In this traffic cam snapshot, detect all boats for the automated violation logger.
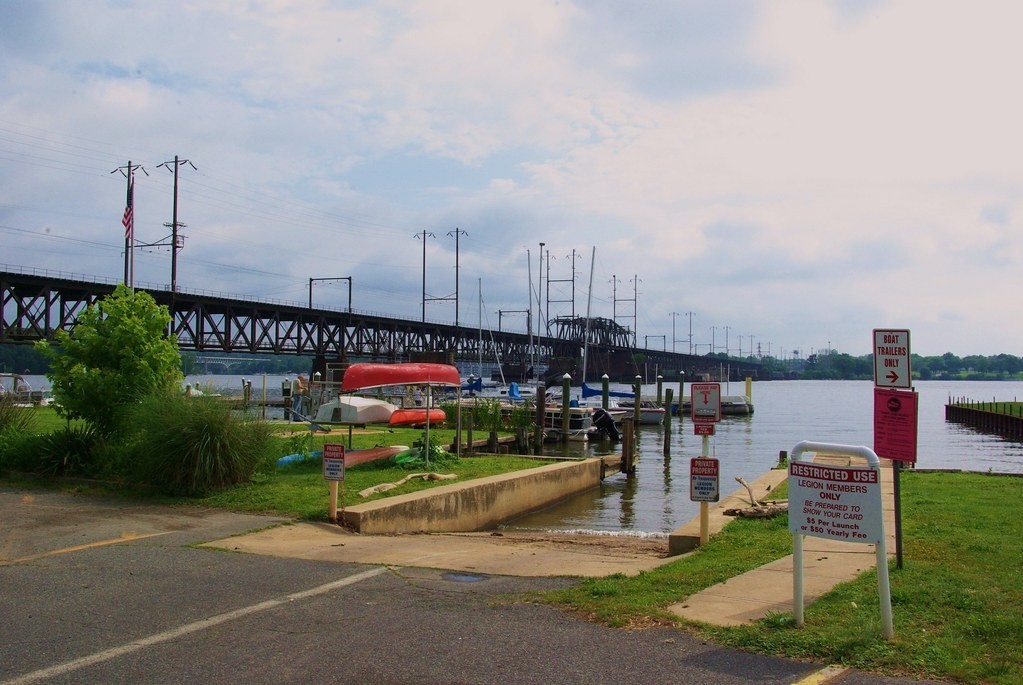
[389,408,447,427]
[337,362,461,395]
[430,246,755,442]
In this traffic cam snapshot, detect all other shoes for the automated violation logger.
[293,419,303,422]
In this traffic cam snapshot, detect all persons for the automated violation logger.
[414,386,425,406]
[293,375,308,421]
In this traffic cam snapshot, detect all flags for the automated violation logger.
[122,176,135,239]
